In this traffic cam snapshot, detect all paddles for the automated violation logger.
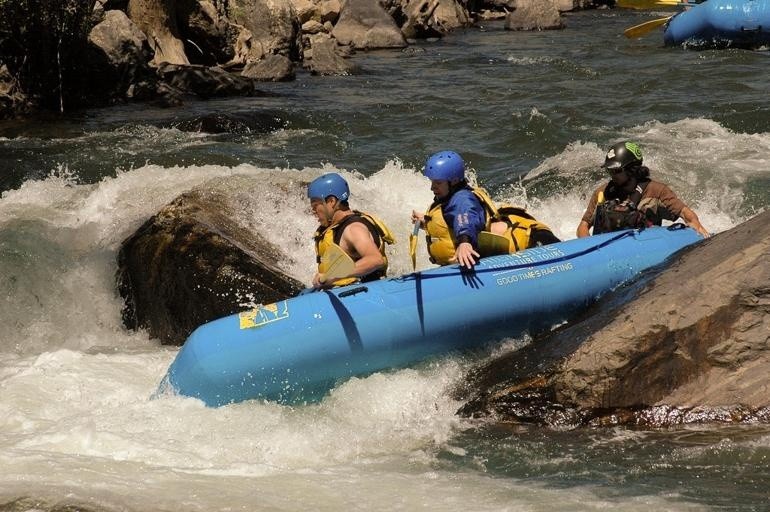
[309,244,355,289]
[408,219,421,269]
[625,17,670,40]
[617,0,697,10]
[478,231,509,256]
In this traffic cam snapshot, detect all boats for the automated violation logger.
[663,1,770,47]
[147,223,704,408]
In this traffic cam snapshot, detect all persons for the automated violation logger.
[305,171,390,292]
[489,201,562,257]
[574,139,712,244]
[411,149,491,269]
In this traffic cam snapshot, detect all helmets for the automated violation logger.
[306,173,352,204]
[424,150,466,183]
[599,139,643,168]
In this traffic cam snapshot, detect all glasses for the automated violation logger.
[607,169,623,177]
